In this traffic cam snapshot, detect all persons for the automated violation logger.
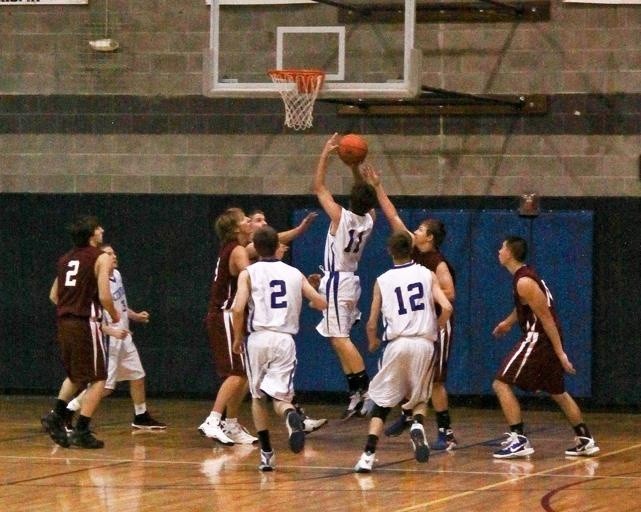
[197,206,290,446]
[354,229,454,473]
[244,210,327,435]
[62,244,168,436]
[490,234,602,459]
[40,212,121,449]
[359,162,459,452]
[231,225,328,471]
[314,130,375,424]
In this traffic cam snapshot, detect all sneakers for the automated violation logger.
[492,433,535,459]
[197,390,457,473]
[42,410,103,450]
[131,412,166,429]
[564,436,601,456]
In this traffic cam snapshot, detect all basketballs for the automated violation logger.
[338,135,367,163]
